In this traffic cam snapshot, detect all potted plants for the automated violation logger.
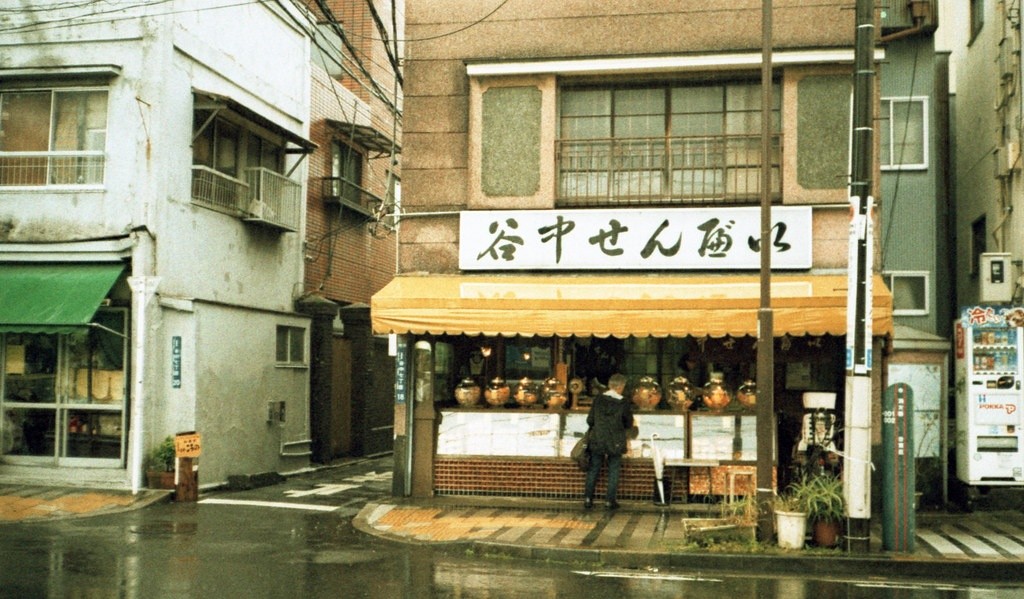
[143,443,160,489]
[773,494,807,550]
[153,435,175,490]
[785,471,844,547]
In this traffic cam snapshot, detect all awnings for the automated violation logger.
[0,262,133,345]
[369,273,897,340]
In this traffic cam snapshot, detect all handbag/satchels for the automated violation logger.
[571,427,591,472]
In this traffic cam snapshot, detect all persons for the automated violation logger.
[582,373,640,511]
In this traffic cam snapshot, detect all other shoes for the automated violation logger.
[605,499,620,510]
[584,497,593,508]
